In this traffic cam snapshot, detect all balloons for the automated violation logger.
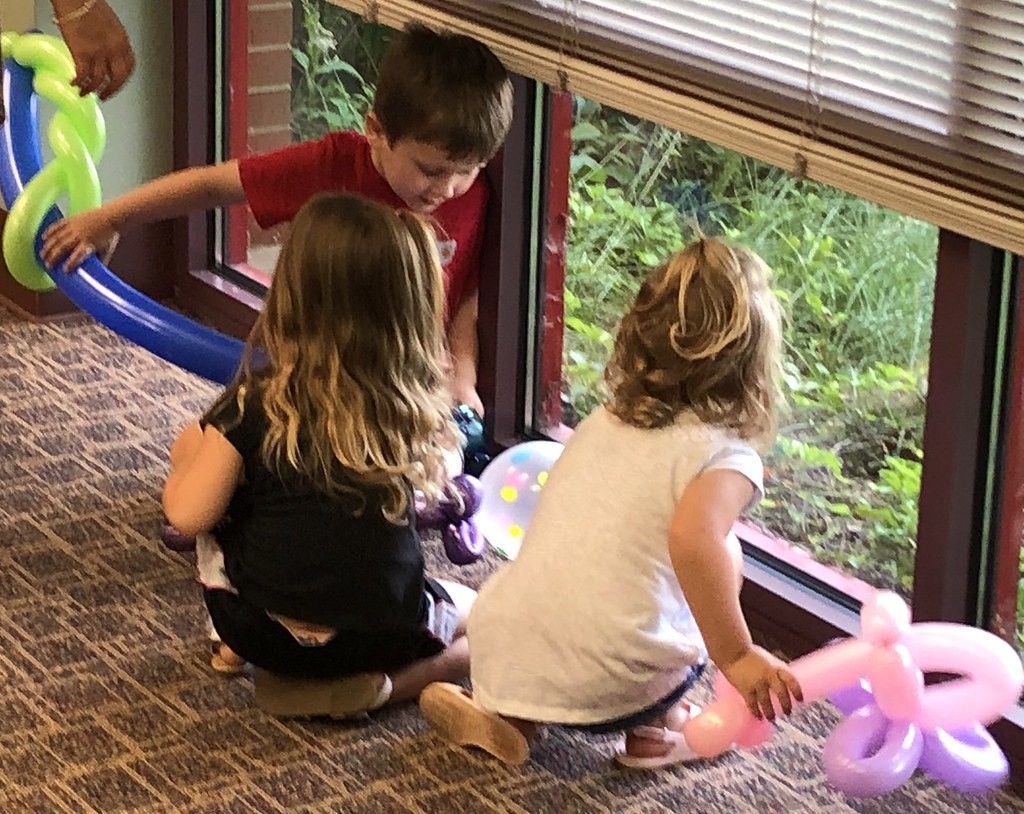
[411,475,484,566]
[473,441,565,562]
[0,29,267,388]
[685,588,1024,799]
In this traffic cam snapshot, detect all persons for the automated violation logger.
[162,192,478,717]
[48,0,136,101]
[418,237,804,769]
[40,26,513,423]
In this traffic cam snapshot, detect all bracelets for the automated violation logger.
[52,0,97,25]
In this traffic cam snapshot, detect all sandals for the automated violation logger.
[210,629,254,675]
[618,700,734,769]
[253,672,393,718]
[420,681,531,766]
